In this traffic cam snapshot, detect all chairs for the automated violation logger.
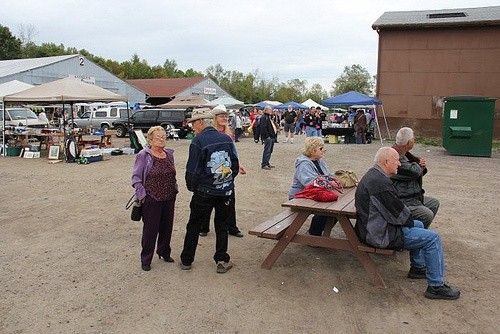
[99,135,111,148]
[74,135,84,150]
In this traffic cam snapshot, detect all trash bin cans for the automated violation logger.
[443,96,496,158]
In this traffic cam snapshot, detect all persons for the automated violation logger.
[231,110,243,143]
[131,126,178,272]
[289,136,341,247]
[31,108,82,124]
[387,127,440,229]
[354,147,460,296]
[180,107,240,273]
[250,107,264,143]
[200,104,246,238]
[259,105,276,170]
[294,106,375,144]
[283,105,297,144]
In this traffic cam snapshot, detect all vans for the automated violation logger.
[94,107,133,128]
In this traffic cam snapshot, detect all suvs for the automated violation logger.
[112,109,188,138]
[0,107,48,135]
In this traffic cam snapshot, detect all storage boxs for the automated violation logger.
[81,149,102,162]
[6,147,21,156]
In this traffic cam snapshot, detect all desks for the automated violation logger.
[42,129,59,136]
[323,128,355,143]
[35,134,65,152]
[260,176,387,290]
[13,133,34,149]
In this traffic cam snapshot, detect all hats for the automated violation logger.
[187,108,214,123]
[210,104,228,115]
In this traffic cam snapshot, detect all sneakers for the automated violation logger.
[425,282,460,299]
[216,260,233,273]
[407,266,427,279]
[180,256,192,269]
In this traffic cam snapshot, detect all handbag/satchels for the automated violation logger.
[335,169,360,188]
[312,173,345,194]
[125,194,142,221]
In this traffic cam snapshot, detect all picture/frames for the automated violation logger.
[48,145,61,160]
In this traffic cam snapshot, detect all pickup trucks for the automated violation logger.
[68,110,118,130]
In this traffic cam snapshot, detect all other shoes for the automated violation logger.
[200,232,207,236]
[262,165,271,170]
[268,164,275,168]
[229,229,243,237]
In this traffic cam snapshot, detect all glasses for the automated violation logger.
[317,147,324,151]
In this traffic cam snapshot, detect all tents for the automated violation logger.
[0,77,131,162]
[157,96,260,111]
[256,91,391,146]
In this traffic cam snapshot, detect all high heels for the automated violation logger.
[158,252,174,262]
[141,264,150,270]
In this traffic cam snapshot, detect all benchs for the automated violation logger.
[248,203,300,238]
